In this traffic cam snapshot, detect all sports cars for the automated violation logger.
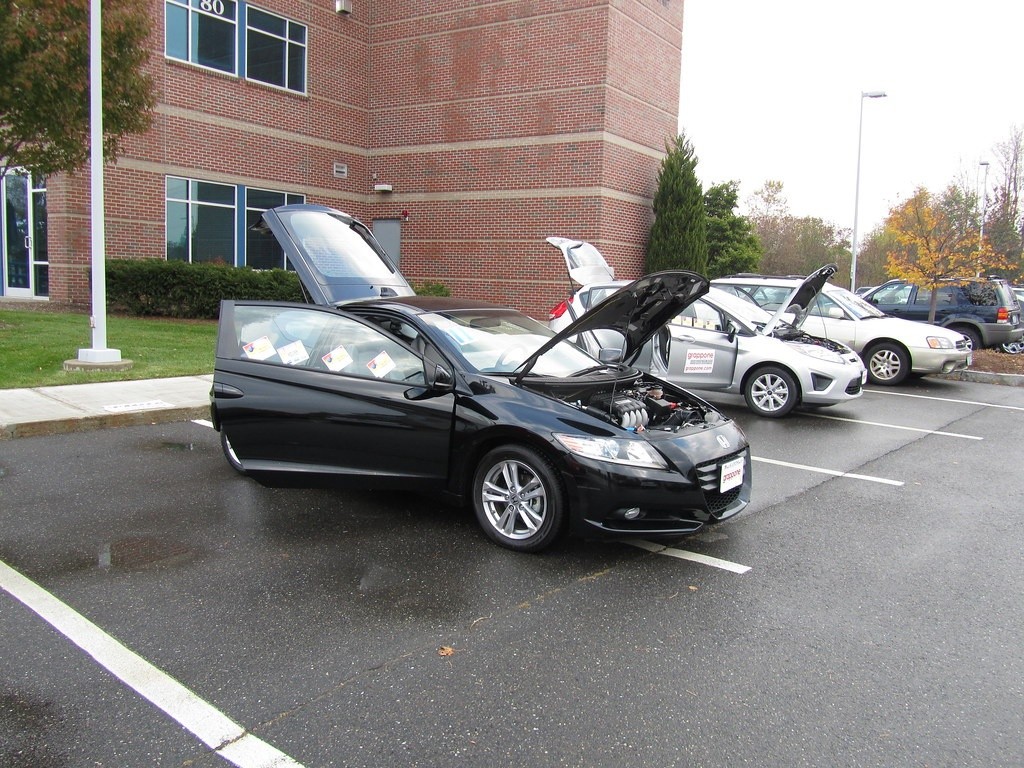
[207,203,754,552]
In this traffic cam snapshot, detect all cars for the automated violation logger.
[542,230,870,418]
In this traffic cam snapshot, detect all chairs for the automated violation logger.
[347,342,399,376]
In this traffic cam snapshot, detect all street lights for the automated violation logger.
[850,90,886,291]
[975,160,990,277]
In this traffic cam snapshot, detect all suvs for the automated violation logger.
[824,276,1024,349]
[704,271,975,384]
